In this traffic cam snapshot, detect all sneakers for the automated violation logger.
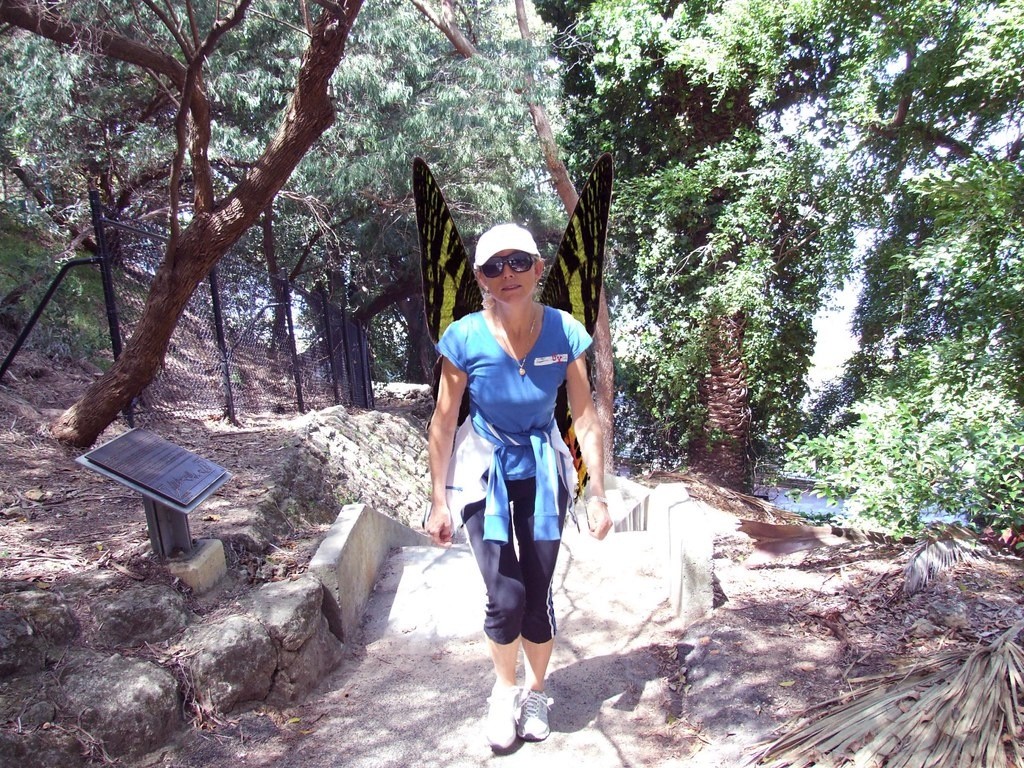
[486,682,520,752]
[517,688,551,741]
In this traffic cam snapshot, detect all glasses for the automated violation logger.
[482,252,533,278]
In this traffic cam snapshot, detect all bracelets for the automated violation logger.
[586,496,609,506]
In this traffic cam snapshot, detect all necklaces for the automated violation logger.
[490,303,538,375]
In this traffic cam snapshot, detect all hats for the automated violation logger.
[475,224,540,267]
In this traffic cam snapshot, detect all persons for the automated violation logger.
[425,223,614,750]
